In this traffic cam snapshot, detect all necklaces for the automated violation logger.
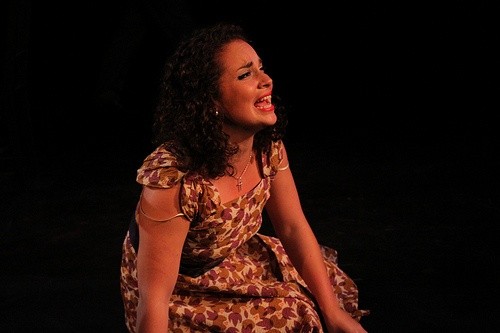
[226,150,254,192]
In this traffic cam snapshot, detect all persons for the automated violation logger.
[119,23,372,333]
